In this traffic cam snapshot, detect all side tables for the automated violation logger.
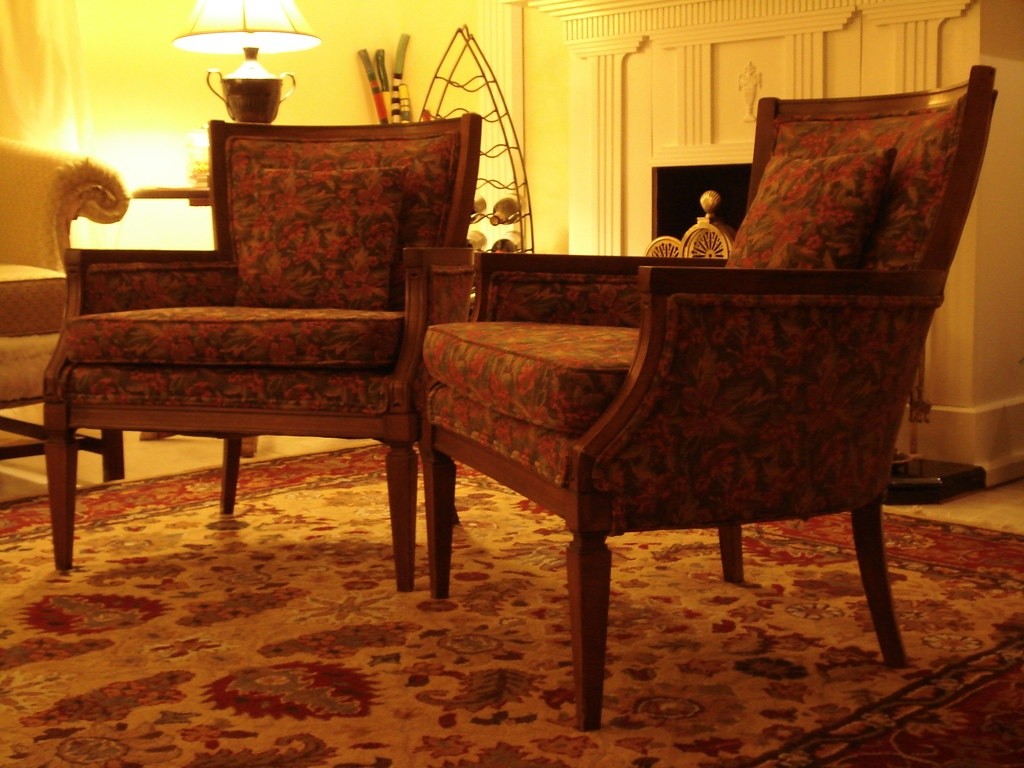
[128,185,258,459]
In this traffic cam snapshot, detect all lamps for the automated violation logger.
[169,0,322,124]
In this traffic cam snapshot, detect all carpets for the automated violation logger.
[0,444,1024,768]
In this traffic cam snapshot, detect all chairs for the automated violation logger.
[43,112,483,593]
[419,63,998,732]
[0,134,126,483]
